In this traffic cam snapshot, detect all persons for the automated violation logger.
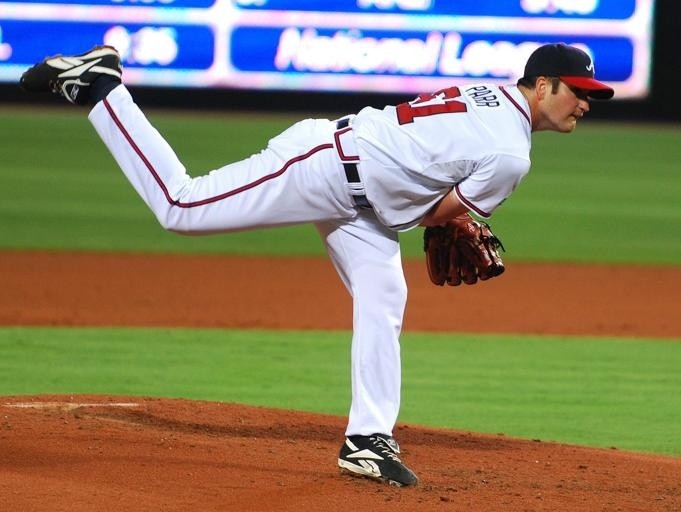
[20,45,614,488]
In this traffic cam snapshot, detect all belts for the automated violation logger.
[335,116,372,212]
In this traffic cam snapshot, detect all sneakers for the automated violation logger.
[18,44,124,107]
[337,433,420,488]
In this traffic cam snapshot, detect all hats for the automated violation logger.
[523,42,615,99]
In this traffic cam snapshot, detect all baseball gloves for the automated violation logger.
[424,212,505,286]
